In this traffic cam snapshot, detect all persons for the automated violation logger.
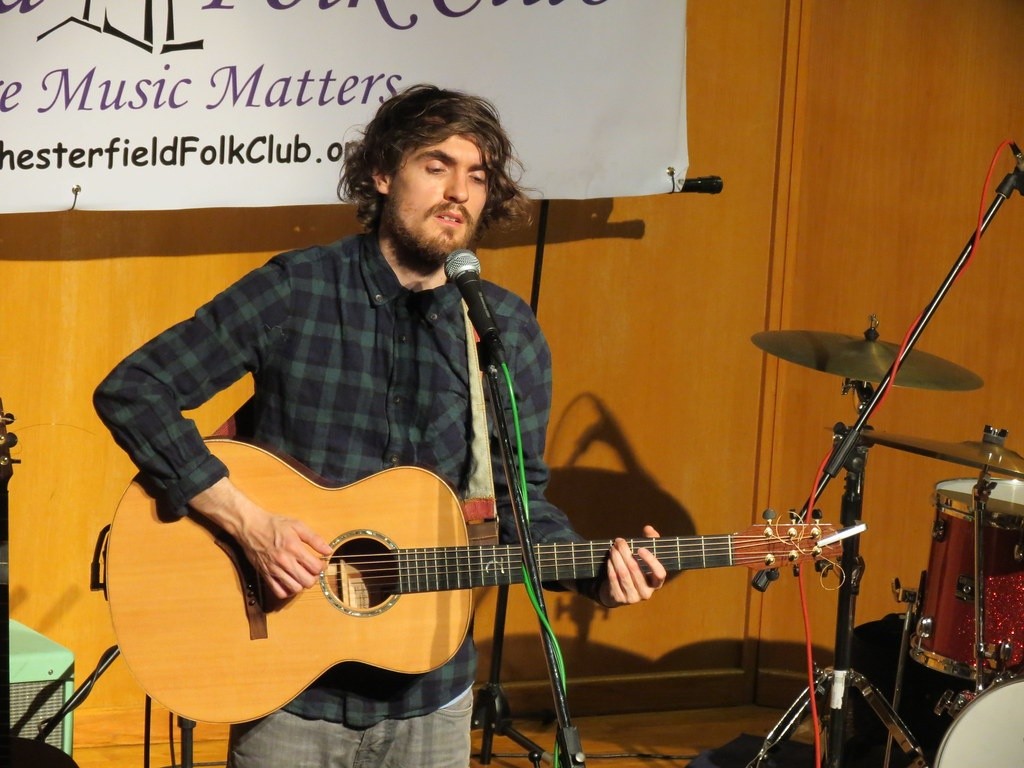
[91,84,665,768]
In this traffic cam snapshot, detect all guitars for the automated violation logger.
[89,435,867,724]
[1,399,82,768]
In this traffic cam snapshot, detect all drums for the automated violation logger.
[931,679,1023,768]
[909,478,1024,683]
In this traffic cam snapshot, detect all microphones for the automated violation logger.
[444,247,508,365]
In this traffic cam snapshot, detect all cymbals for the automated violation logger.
[749,329,984,393]
[824,424,1023,480]
